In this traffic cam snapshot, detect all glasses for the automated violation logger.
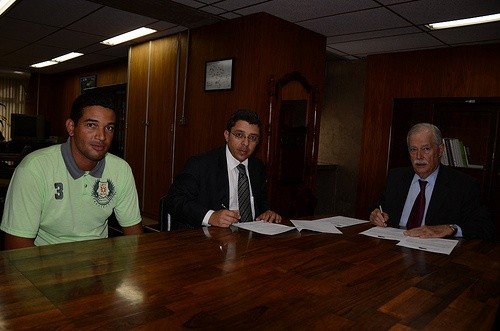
[228,130,260,143]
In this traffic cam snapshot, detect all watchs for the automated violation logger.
[449,225,457,236]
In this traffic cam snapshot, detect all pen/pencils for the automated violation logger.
[222,204,243,223]
[379,205,387,228]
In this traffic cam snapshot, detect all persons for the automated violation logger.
[0,91,142,250]
[368,123,496,241]
[164,108,282,228]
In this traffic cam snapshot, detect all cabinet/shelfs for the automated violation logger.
[77,82,126,157]
[388,96,500,204]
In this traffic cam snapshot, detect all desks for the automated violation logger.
[0,229,500,331]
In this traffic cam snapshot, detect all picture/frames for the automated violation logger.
[203,57,236,91]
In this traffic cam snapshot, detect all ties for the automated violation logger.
[406,179,428,232]
[237,164,253,222]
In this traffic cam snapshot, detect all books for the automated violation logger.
[441,138,469,168]
